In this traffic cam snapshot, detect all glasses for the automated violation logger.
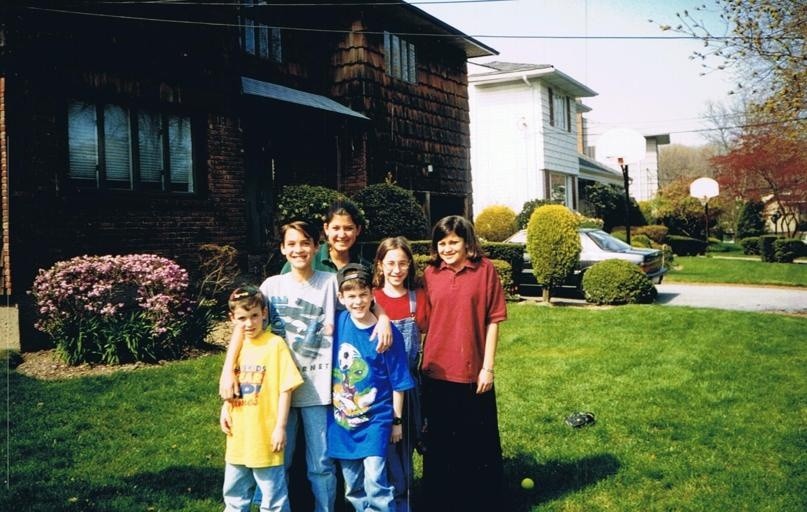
[381,260,412,268]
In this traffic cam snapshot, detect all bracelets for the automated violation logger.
[482,368,495,373]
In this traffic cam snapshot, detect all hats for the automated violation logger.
[336,263,375,308]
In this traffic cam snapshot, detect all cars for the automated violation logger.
[499,224,668,297]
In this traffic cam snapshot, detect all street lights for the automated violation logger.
[689,175,721,236]
[594,128,651,244]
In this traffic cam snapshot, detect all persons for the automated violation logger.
[219,284,304,512]
[327,263,414,511]
[369,237,427,493]
[218,221,393,512]
[418,216,507,507]
[280,202,374,285]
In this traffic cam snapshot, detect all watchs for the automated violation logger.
[393,418,403,425]
